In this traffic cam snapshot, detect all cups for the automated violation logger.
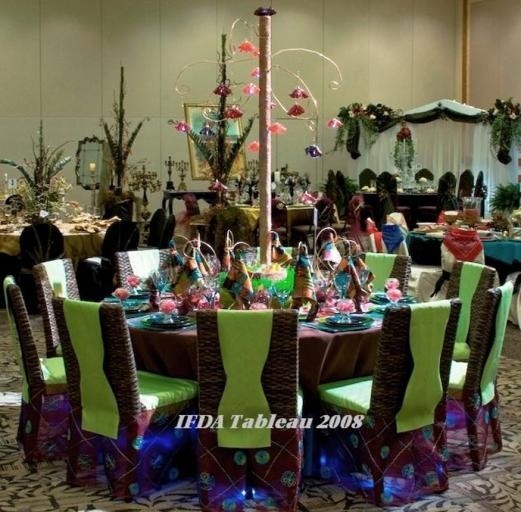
[358,270,369,287]
[334,273,352,298]
[199,278,218,309]
[150,269,170,302]
[274,280,293,309]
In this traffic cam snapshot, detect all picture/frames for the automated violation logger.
[184,103,248,180]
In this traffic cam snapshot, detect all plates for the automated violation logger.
[324,315,374,328]
[128,291,150,299]
[371,293,416,304]
[376,305,409,315]
[112,302,151,315]
[140,314,196,329]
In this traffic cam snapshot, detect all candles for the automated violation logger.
[90,163,96,171]
